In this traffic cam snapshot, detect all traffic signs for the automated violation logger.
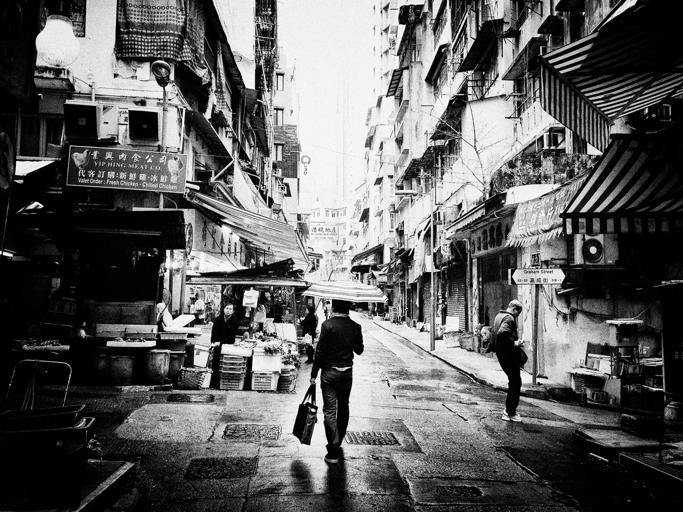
[507,268,566,286]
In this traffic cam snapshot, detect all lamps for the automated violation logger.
[148,56,174,212]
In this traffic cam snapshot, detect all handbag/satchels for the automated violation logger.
[486,333,498,354]
[292,384,318,445]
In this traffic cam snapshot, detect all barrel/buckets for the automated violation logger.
[193,345,209,368]
[170,349,187,379]
[146,348,170,378]
[665,403,682,439]
[570,369,585,393]
[110,353,132,380]
[584,373,604,400]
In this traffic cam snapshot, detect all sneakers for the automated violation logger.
[324,451,339,463]
[502,407,523,422]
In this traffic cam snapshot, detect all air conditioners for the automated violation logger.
[565,230,620,268]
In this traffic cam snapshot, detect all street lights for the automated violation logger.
[148,59,170,208]
[395,188,437,351]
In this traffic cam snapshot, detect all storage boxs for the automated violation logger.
[181,334,297,394]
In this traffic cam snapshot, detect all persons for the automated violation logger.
[299,305,316,364]
[321,300,332,322]
[492,299,526,422]
[308,300,365,466]
[265,297,283,324]
[210,301,237,389]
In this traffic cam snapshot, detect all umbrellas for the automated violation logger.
[298,276,389,306]
